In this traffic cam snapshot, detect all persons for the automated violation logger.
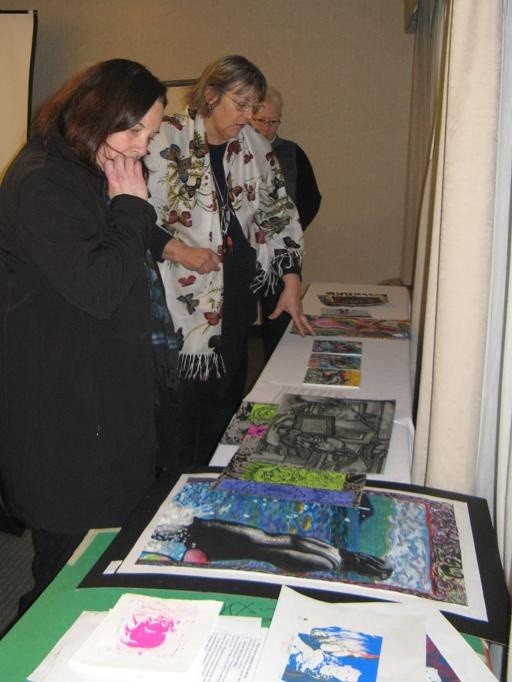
[222,83,327,410]
[1,57,180,606]
[143,51,318,463]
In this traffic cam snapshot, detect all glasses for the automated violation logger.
[209,83,265,114]
[252,117,282,127]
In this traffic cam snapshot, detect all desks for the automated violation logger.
[150,279,417,682]
